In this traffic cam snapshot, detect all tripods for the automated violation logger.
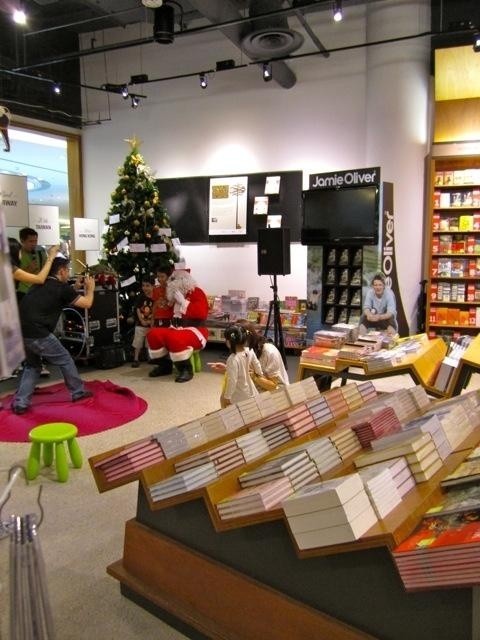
[263,275,288,371]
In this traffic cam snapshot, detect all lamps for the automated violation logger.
[332,0,344,26]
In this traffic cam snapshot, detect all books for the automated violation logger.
[435,168,480,184]
[359,332,428,374]
[299,323,359,370]
[431,282,480,301]
[433,214,480,230]
[433,190,480,206]
[432,257,480,276]
[93,396,262,550]
[263,396,390,550]
[391,392,480,595]
[210,295,306,324]
[432,235,480,253]
[428,331,478,347]
[430,307,480,325]
[428,347,469,392]
[289,372,429,395]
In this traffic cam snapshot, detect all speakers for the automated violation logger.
[257,227,291,276]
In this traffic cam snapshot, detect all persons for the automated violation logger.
[145,260,210,383]
[7,238,60,294]
[358,276,399,332]
[18,227,47,294]
[130,275,156,368]
[207,323,289,395]
[221,325,263,410]
[10,257,95,416]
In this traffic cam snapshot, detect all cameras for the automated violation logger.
[80,269,95,283]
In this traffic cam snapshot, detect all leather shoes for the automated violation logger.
[149,366,172,377]
[14,405,26,414]
[175,369,193,382]
[72,391,92,401]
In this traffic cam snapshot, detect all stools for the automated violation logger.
[22,422,83,484]
[180,347,205,376]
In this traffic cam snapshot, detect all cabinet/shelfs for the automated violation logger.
[87,376,480,639]
[296,325,479,396]
[321,241,365,332]
[427,152,480,338]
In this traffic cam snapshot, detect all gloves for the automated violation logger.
[166,289,174,305]
[174,290,185,304]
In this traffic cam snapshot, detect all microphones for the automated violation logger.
[31,248,36,261]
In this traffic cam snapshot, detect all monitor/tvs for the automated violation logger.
[301,181,379,246]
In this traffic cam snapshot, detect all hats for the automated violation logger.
[172,262,191,273]
